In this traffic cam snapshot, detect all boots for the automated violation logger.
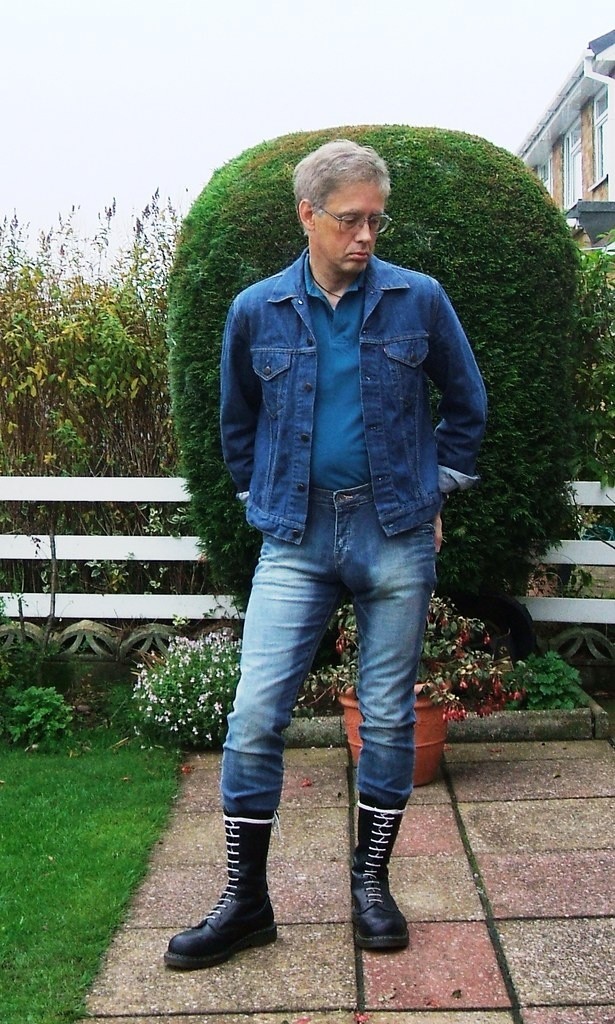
[163,810,281,968]
[351,792,410,948]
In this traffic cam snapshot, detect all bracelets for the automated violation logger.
[442,494,450,503]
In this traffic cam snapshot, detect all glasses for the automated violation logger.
[314,203,393,235]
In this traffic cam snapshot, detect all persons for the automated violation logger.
[163,138,487,967]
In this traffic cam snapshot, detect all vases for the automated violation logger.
[338,694,447,787]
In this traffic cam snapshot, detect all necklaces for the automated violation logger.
[308,260,341,298]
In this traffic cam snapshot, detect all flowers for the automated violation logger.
[127,630,240,744]
[302,594,524,721]
